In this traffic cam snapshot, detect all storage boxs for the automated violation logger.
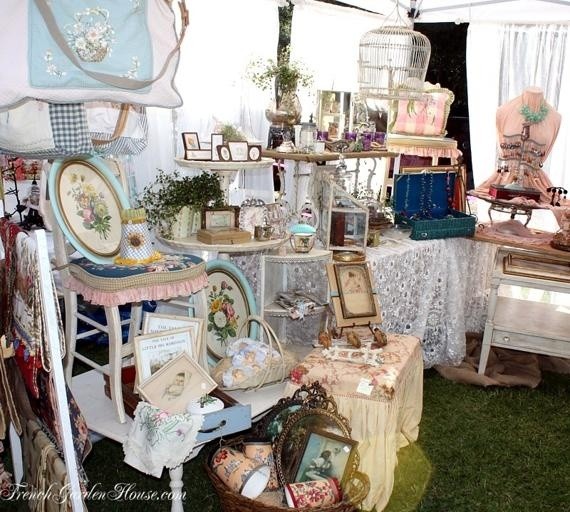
[393,173,476,241]
[1,2,190,159]
[185,149,211,161]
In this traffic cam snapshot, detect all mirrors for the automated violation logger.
[281,414,344,481]
[267,405,301,442]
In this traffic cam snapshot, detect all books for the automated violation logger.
[132,311,218,413]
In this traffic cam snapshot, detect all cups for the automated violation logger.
[289,232,316,254]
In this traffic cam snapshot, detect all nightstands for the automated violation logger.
[477,246,569,376]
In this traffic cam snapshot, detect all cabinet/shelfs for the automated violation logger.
[261,249,333,361]
[231,220,570,370]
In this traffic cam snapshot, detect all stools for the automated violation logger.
[291,330,423,512]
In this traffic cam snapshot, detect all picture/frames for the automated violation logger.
[49,153,130,265]
[133,312,204,394]
[136,351,218,415]
[276,391,360,486]
[189,260,258,367]
[211,133,223,161]
[182,132,201,149]
[134,326,198,403]
[217,145,230,162]
[248,145,261,161]
[202,206,240,229]
[228,140,248,162]
[256,380,328,442]
[289,426,358,502]
[326,261,382,328]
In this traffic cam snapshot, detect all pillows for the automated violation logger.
[387,85,455,137]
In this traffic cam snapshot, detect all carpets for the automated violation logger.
[0,368,570,512]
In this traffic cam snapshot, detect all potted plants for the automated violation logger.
[134,168,227,239]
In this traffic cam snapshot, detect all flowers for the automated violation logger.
[248,45,316,91]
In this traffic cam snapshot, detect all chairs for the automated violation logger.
[71,360,299,511]
[51,154,209,426]
[382,79,463,204]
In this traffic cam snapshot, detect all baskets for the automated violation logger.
[206,444,370,512]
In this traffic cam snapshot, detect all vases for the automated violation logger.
[266,92,303,151]
